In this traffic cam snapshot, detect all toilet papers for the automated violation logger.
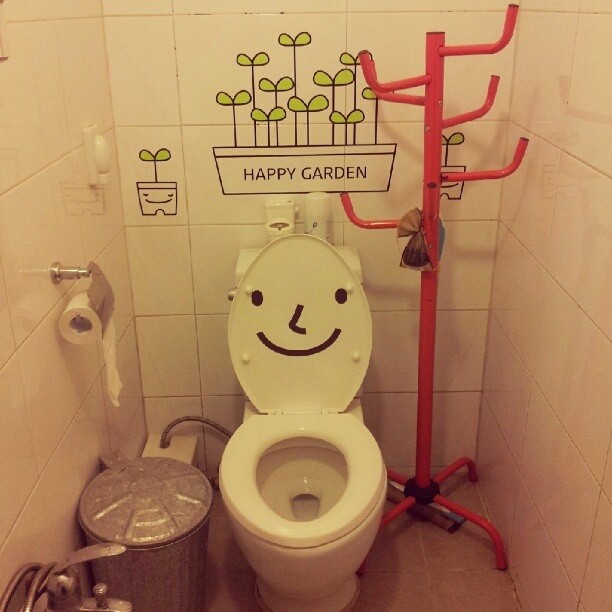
[58,292,123,411]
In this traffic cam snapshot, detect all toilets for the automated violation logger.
[218,233,388,612]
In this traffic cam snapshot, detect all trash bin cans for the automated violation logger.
[79,448,212,612]
